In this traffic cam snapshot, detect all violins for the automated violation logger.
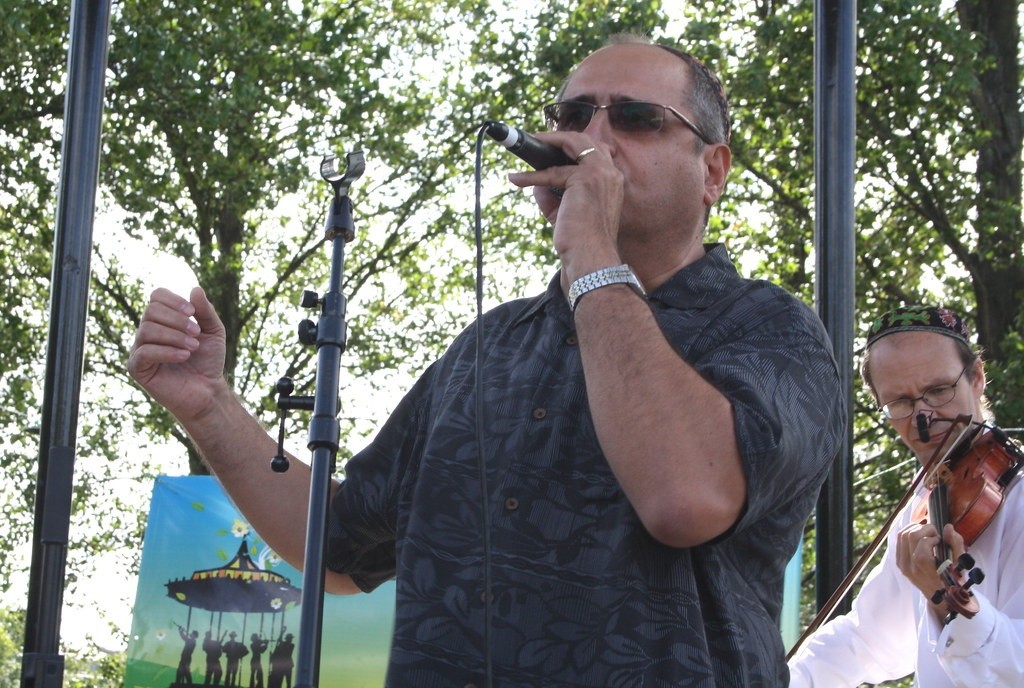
[910,426,1020,625]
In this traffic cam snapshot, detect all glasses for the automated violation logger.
[879,359,973,420]
[544,101,712,146]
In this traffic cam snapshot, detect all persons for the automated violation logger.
[127,36,847,688]
[786,306,1023,688]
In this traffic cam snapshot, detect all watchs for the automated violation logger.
[567,264,646,311]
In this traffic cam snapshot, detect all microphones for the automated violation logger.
[483,121,580,198]
[917,413,931,443]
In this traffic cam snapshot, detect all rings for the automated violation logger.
[575,148,598,164]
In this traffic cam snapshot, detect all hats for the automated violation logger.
[866,305,971,346]
